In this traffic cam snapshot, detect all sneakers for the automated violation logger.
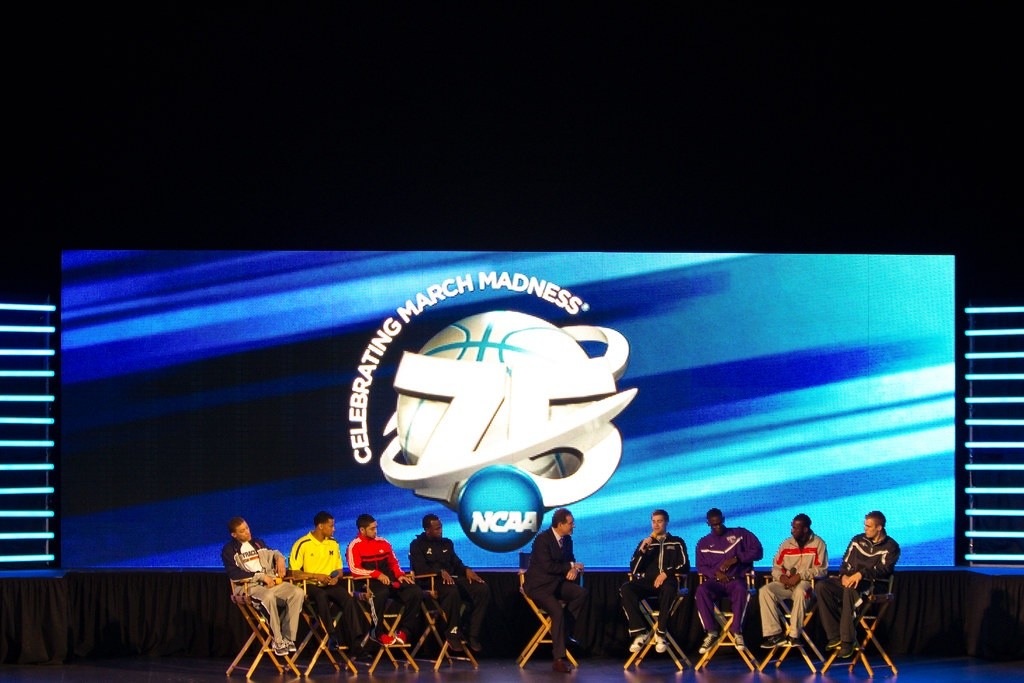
[446,632,462,652]
[655,633,666,652]
[327,638,340,651]
[378,634,396,645]
[272,642,289,656]
[760,634,786,648]
[396,631,407,644]
[283,638,296,651]
[826,637,841,650]
[350,648,372,660]
[629,631,648,653]
[469,636,481,651]
[841,638,857,658]
[698,635,717,654]
[787,637,802,646]
[734,633,744,650]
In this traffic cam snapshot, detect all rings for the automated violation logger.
[787,586,788,587]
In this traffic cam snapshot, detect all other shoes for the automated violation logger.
[552,659,571,672]
[567,629,580,642]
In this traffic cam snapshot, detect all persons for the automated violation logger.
[694,509,763,654]
[815,511,900,657]
[623,510,690,652]
[288,512,372,659]
[524,509,588,672]
[410,513,489,651]
[758,514,830,648]
[223,517,304,656]
[346,514,422,645]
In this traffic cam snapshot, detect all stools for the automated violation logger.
[516,552,583,668]
[226,552,478,679]
[623,569,898,678]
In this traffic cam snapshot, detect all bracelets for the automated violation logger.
[734,556,737,558]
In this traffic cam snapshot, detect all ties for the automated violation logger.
[560,539,563,548]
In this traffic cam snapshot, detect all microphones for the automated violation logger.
[717,577,736,581]
[388,581,401,589]
[643,531,657,549]
[259,579,283,586]
[316,570,338,586]
[785,567,797,589]
